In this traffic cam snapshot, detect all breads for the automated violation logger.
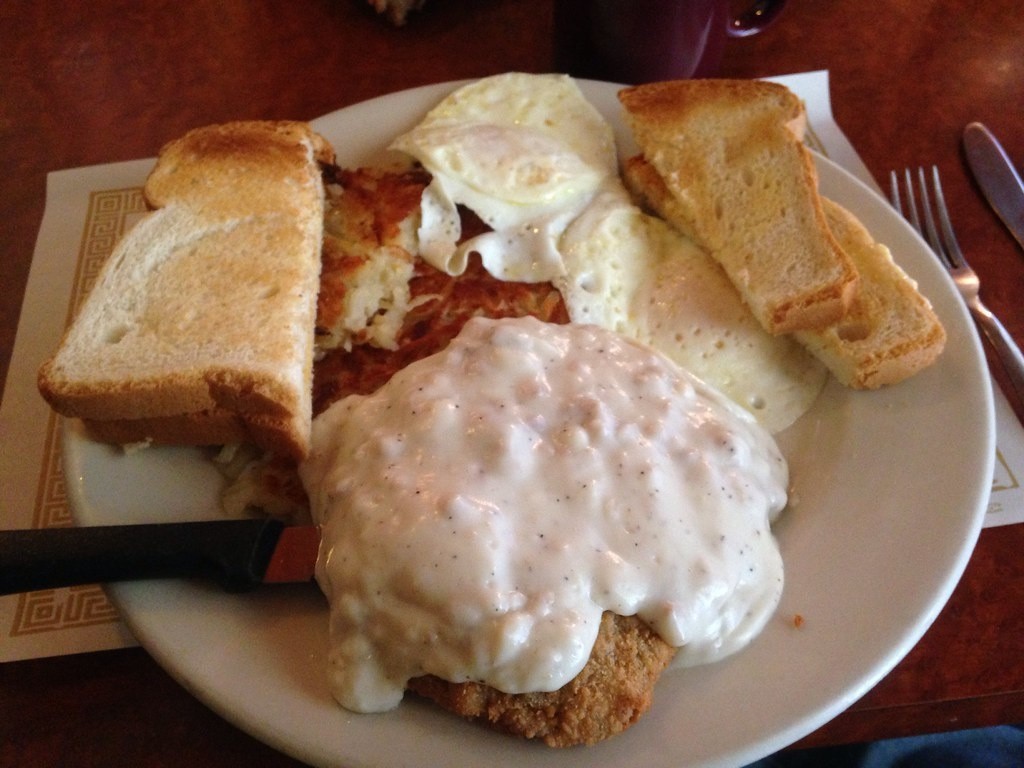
[35,79,945,458]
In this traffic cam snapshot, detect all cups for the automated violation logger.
[552,0,713,88]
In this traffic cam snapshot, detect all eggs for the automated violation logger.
[301,73,827,713]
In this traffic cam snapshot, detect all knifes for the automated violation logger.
[1,520,322,595]
[963,122,1024,250]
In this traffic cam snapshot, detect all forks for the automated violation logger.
[888,167,1024,404]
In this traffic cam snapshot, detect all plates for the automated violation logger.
[63,73,995,768]
